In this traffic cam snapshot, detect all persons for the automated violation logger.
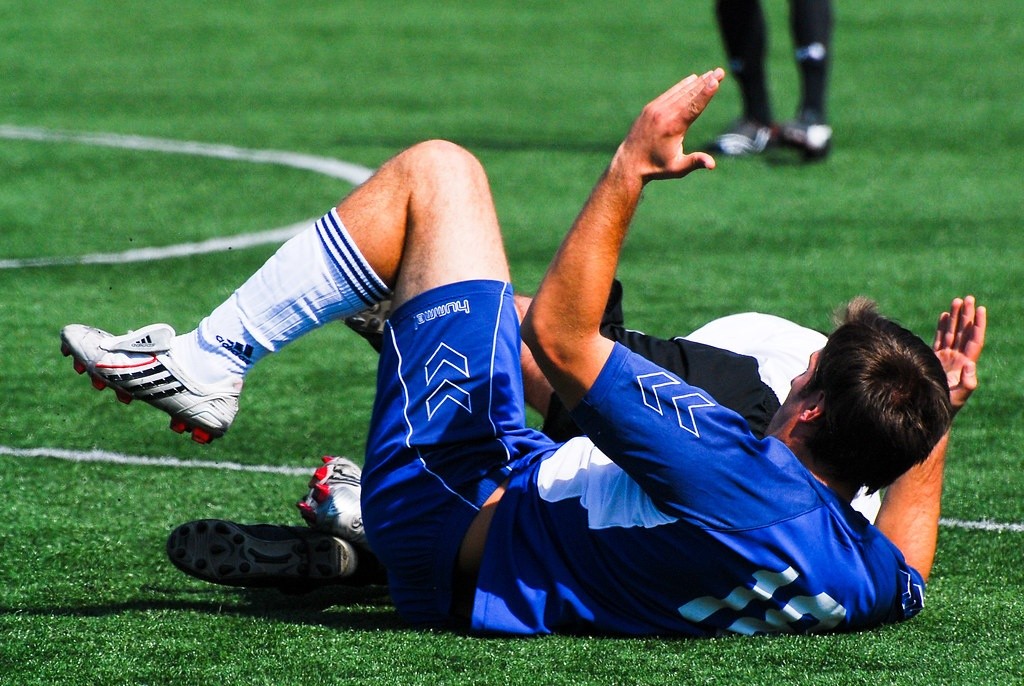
[299,280,882,588]
[714,0,834,162]
[59,67,987,640]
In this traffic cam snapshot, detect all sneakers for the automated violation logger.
[166,517,362,584]
[341,287,396,354]
[295,454,365,544]
[59,322,243,445]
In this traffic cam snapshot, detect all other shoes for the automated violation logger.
[781,119,833,155]
[707,121,780,156]
